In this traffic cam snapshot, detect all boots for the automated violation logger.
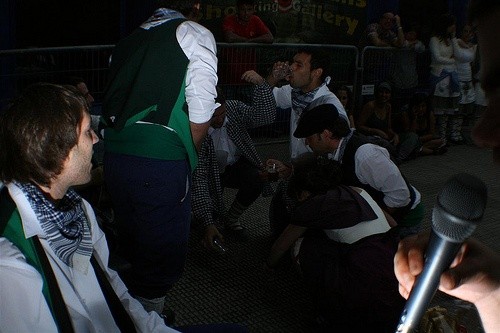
[223,196,249,240]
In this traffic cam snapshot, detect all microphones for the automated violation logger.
[394,173,486,333]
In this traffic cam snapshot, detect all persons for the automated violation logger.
[222,0,273,106]
[267,104,425,244]
[0,81,247,333]
[264,156,416,322]
[392,0,500,333]
[266,0,491,160]
[265,44,355,161]
[55,78,112,228]
[189,68,277,250]
[97,1,218,328]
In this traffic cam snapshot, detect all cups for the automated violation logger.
[214,238,230,258]
[273,64,292,80]
[266,163,278,181]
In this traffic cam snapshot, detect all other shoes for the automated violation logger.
[451,138,464,146]
[160,309,175,326]
[439,144,448,154]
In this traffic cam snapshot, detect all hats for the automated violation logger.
[293,104,340,138]
[377,82,392,90]
